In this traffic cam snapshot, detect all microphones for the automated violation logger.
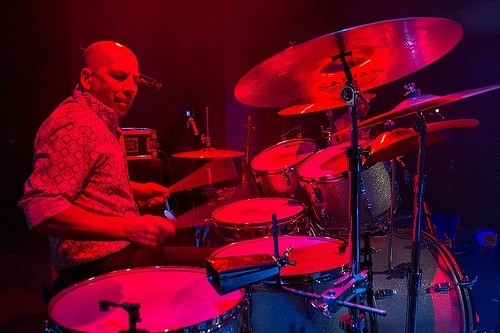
[187,113,198,136]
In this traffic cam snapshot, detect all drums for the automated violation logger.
[359,226,477,333]
[212,198,309,243]
[251,138,318,197]
[45,266,250,333]
[298,139,400,234]
[207,235,350,333]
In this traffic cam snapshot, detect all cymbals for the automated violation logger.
[234,17,463,107]
[325,82,497,132]
[362,118,478,165]
[172,147,245,159]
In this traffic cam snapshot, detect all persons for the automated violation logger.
[18,40,217,286]
[331,94,397,236]
[159,105,211,180]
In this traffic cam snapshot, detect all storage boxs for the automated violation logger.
[121,128,157,160]
